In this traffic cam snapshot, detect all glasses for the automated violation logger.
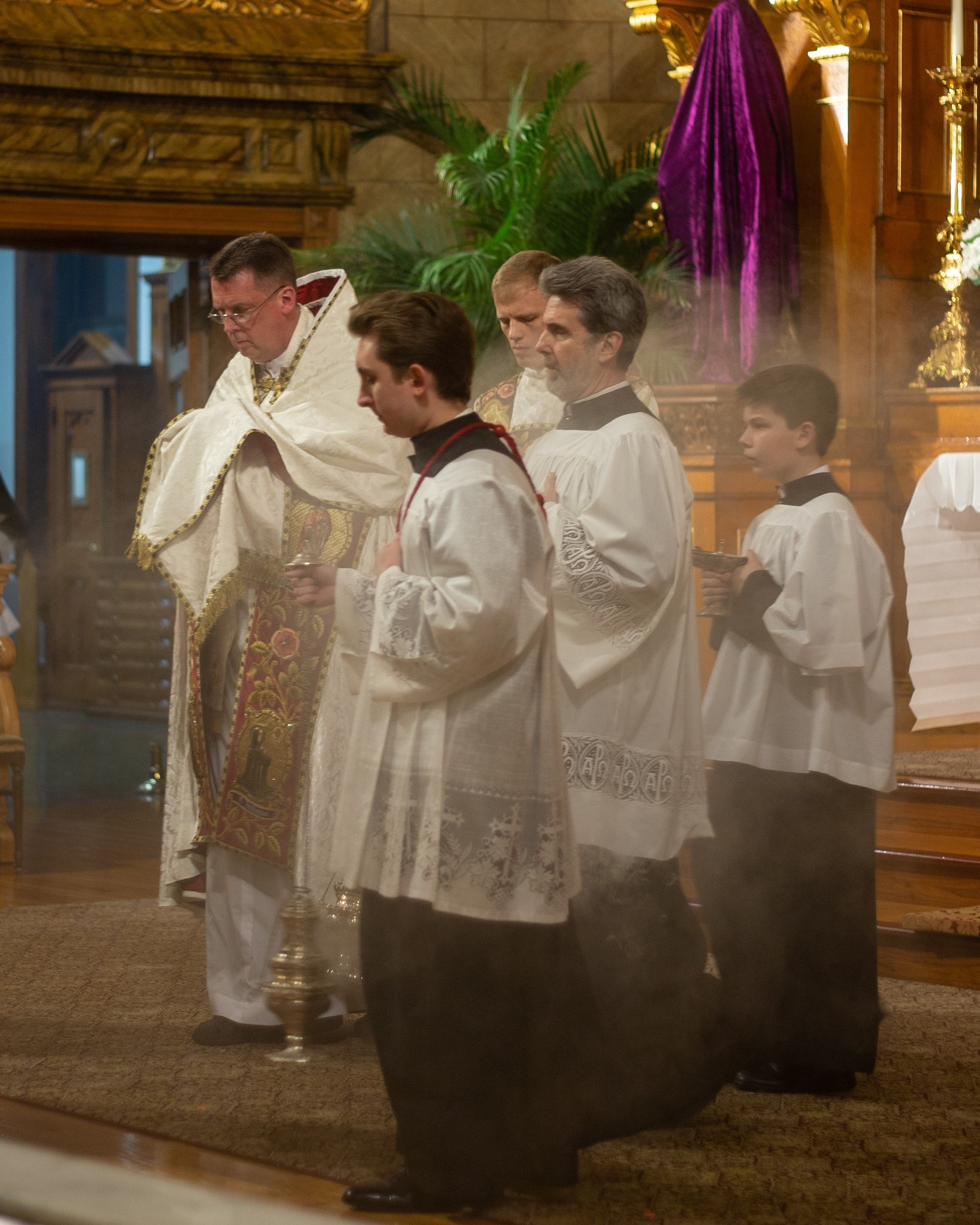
[207,285,286,324]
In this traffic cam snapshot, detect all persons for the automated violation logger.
[189,224,412,1051]
[283,287,599,1211]
[695,361,893,1097]
[463,247,662,480]
[521,254,720,1147]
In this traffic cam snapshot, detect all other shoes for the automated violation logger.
[737,1071,857,1093]
[191,1014,344,1045]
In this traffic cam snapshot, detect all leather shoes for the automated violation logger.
[343,1165,463,1215]
